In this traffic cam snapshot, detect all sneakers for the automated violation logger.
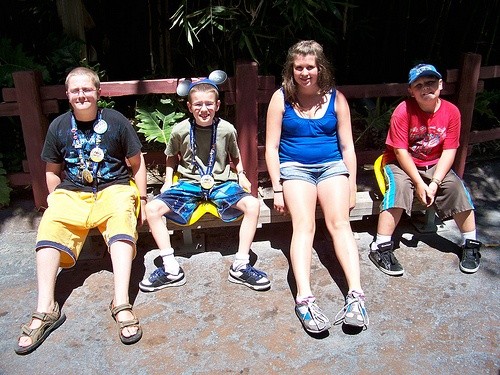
[138,266,187,292]
[295,296,332,333]
[341,291,366,328]
[458,240,482,272]
[368,241,404,276]
[228,263,270,290]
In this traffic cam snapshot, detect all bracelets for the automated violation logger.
[274,190,283,193]
[430,177,441,187]
[236,169,247,177]
[139,194,148,202]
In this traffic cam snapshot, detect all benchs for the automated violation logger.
[76,171,450,261]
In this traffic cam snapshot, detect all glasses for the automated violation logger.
[69,88,97,95]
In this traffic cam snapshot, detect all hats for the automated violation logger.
[408,64,442,85]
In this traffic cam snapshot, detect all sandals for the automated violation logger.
[14,303,67,353]
[109,300,142,344]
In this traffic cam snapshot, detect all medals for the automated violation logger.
[81,168,93,184]
[200,174,214,190]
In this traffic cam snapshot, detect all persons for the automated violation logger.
[10,66,150,356]
[264,39,371,333]
[135,80,273,292]
[368,64,484,277]
